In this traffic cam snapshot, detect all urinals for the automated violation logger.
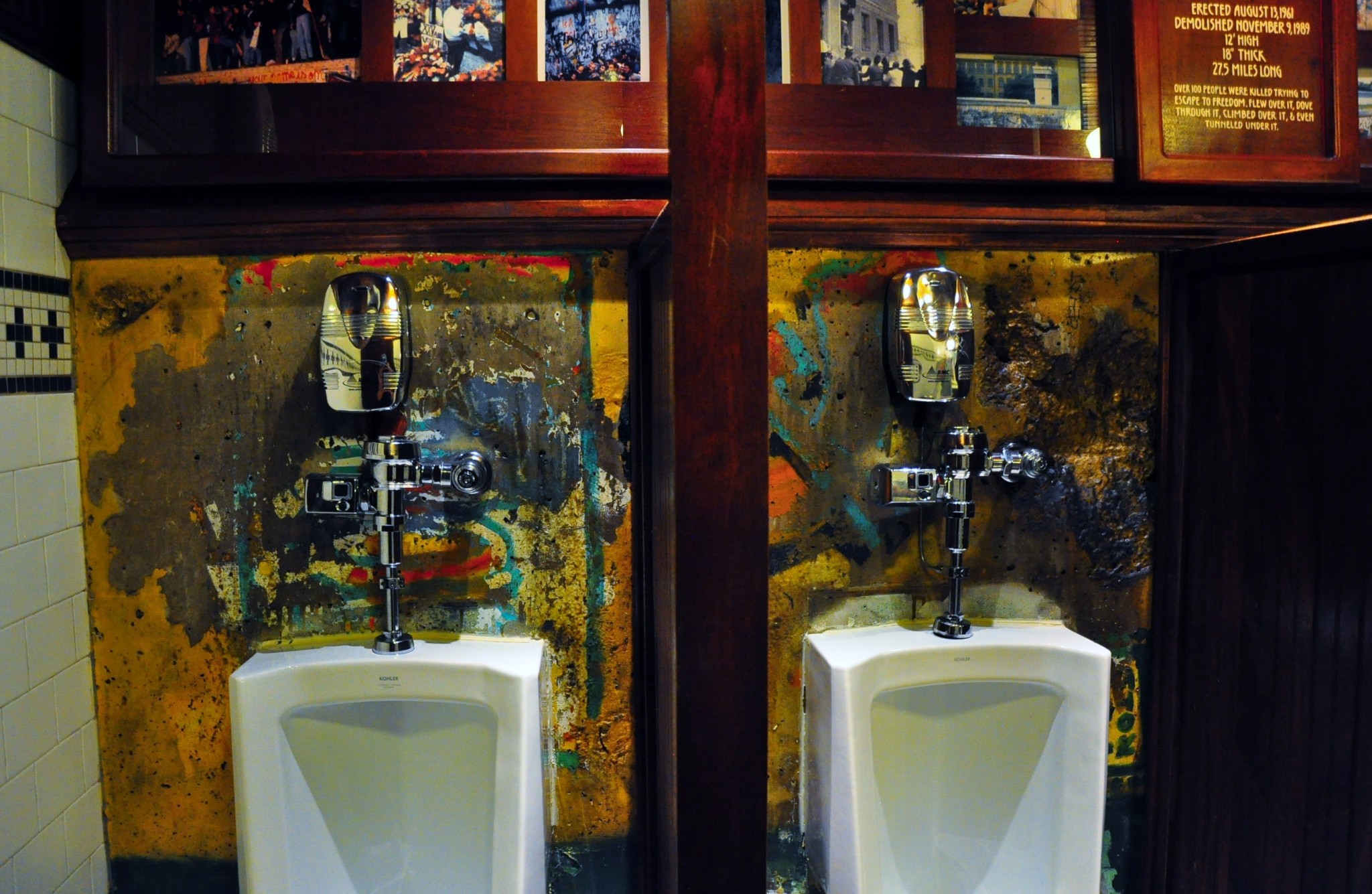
[223,631,550,894]
[803,618,1110,893]
